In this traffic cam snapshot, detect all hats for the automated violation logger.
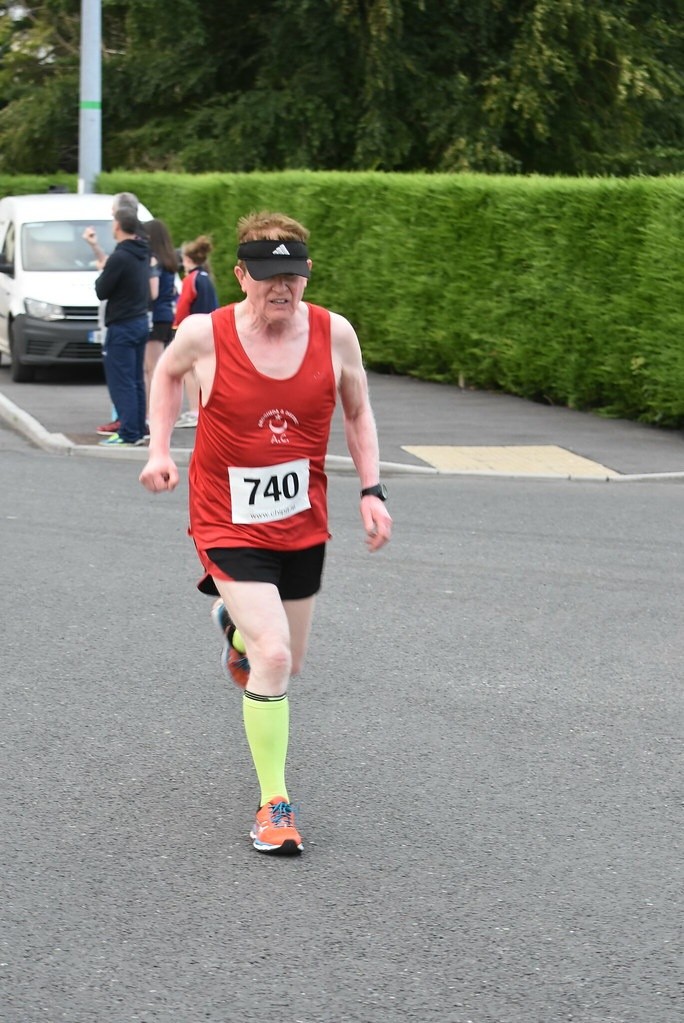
[237,241,310,280]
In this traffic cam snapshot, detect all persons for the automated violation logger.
[82,192,180,437]
[173,236,217,428]
[139,210,393,852]
[94,207,150,447]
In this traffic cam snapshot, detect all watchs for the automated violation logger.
[360,483,389,502]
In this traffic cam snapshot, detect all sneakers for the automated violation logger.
[99,433,145,447]
[96,419,150,439]
[251,796,304,854]
[211,598,250,691]
[174,412,198,428]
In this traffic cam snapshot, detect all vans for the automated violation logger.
[0,195,184,383]
